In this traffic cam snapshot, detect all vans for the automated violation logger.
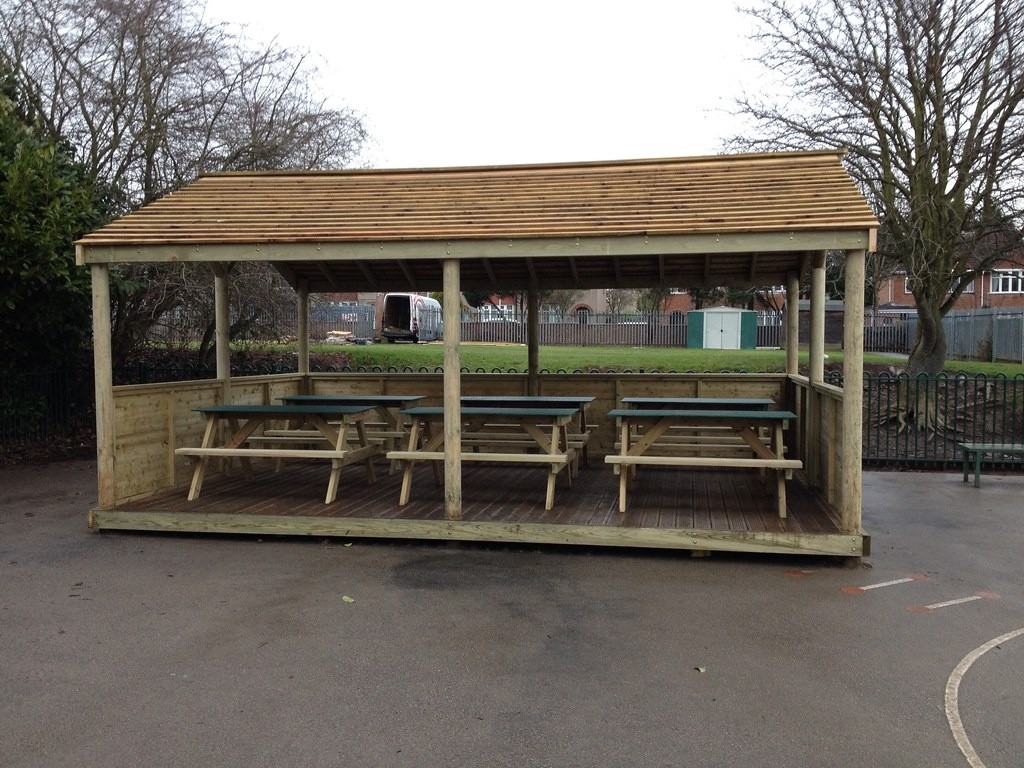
[379,291,445,343]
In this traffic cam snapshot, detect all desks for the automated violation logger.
[397,406,580,510]
[275,395,428,474]
[186,405,387,505]
[621,396,777,480]
[605,409,803,518]
[462,395,597,477]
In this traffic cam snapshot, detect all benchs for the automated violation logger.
[265,430,407,438]
[304,422,427,428]
[244,436,388,445]
[462,432,589,440]
[464,423,599,430]
[618,435,772,444]
[386,450,569,463]
[958,442,1024,488]
[629,426,769,432]
[174,447,349,458]
[424,439,585,449]
[605,455,802,468]
[614,443,787,453]
